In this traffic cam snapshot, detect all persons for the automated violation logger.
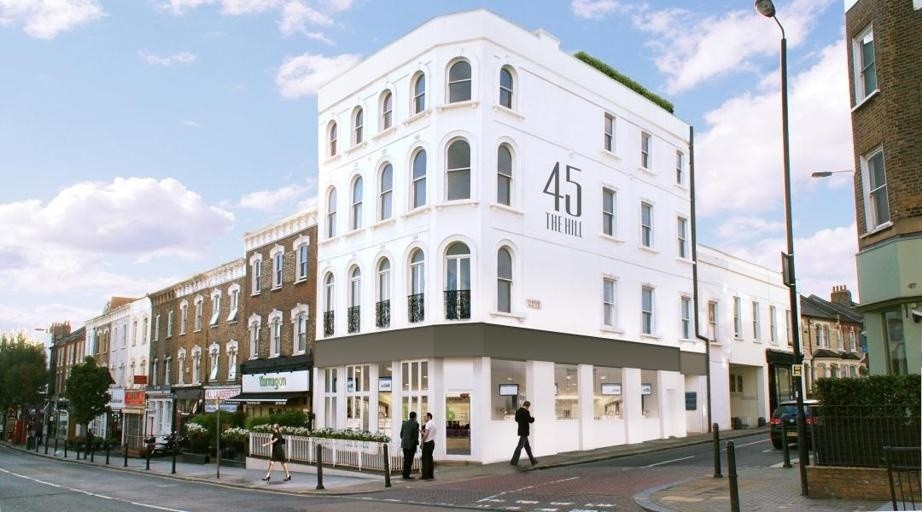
[510,400,539,467]
[400,411,419,479]
[418,413,436,480]
[262,424,293,483]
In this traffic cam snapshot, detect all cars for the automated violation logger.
[769,399,821,450]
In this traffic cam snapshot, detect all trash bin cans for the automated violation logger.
[733,417,741,429]
[759,417,765,426]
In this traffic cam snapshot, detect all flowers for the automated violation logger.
[221,426,249,449]
[185,423,209,441]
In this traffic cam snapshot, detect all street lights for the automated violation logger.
[756,0,811,500]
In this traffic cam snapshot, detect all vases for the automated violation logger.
[190,437,209,453]
[228,445,245,459]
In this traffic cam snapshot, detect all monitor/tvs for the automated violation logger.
[641,383,651,395]
[379,376,392,392]
[499,384,519,396]
[554,383,558,395]
[601,383,622,396]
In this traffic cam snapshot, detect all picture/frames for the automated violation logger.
[378,375,391,392]
